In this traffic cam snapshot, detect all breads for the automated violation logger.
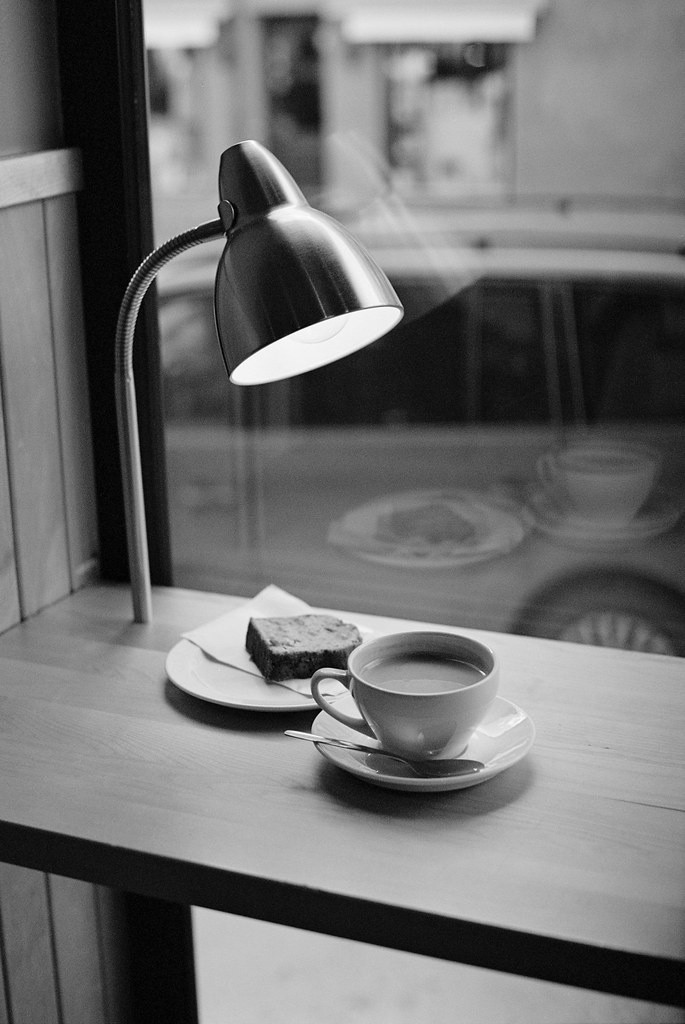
[374,504,476,549]
[245,616,361,681]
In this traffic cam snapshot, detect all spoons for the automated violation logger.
[284,730,485,778]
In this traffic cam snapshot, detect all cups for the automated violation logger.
[533,443,659,523]
[310,631,498,765]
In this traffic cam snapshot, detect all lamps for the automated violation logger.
[115,141,405,627]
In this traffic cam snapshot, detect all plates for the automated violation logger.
[518,486,685,552]
[312,684,537,792]
[167,596,381,712]
[339,491,527,571]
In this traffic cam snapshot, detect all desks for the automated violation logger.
[0,584,685,1010]
